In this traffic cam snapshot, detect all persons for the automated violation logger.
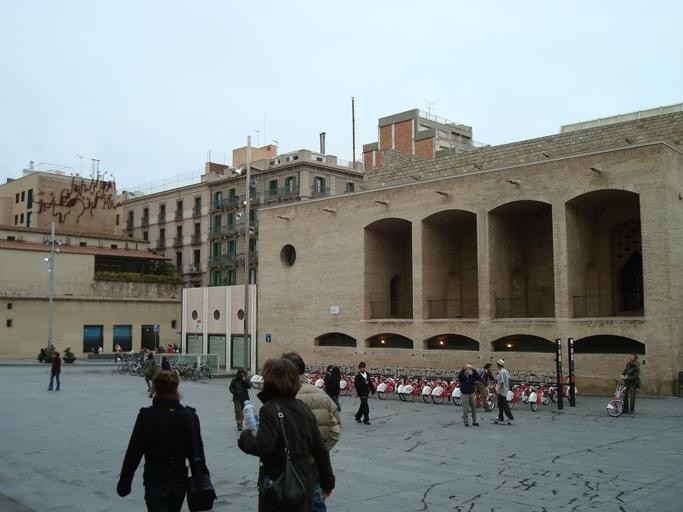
[354,362,376,425]
[114,343,123,363]
[457,363,482,426]
[133,344,174,397]
[116,369,210,512]
[494,358,514,425]
[48,352,61,391]
[90,344,102,355]
[475,363,495,412]
[620,354,640,415]
[229,352,342,512]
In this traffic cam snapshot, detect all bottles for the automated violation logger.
[240,400,260,442]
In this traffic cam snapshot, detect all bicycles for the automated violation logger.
[118,356,211,381]
[606,379,625,417]
[476,369,579,412]
[306,362,463,406]
[250,375,264,389]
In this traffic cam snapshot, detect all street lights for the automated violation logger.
[235,136,254,372]
[47,222,62,348]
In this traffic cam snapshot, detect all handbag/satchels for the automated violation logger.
[636,378,642,390]
[259,452,314,512]
[188,473,216,511]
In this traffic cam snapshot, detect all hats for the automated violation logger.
[496,358,505,366]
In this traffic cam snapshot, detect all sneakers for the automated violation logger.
[465,416,513,426]
[238,426,243,430]
[355,418,370,424]
